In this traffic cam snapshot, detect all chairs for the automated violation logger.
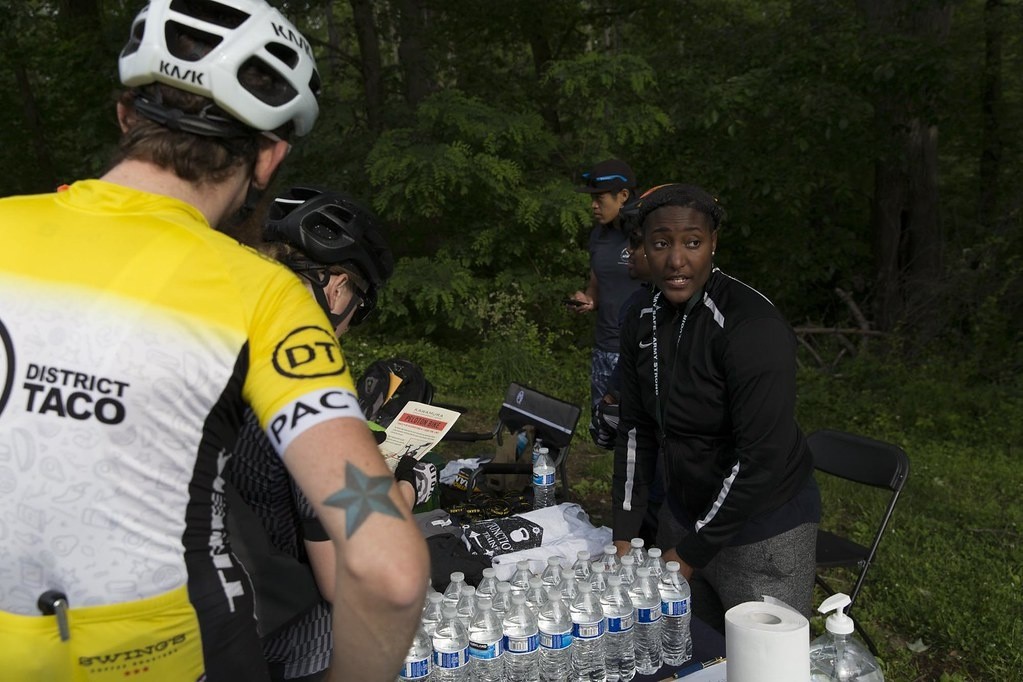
[438,379,582,502]
[806,428,910,656]
[356,361,434,405]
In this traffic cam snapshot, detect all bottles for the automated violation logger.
[532,447,557,510]
[807,592,885,682]
[394,537,693,682]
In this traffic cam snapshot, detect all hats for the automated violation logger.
[574,159,635,193]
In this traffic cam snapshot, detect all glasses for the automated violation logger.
[625,233,643,250]
[322,269,378,311]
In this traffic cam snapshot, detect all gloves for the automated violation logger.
[589,399,621,451]
[395,455,438,511]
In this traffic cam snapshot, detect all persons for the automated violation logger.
[566,159,647,452]
[589,184,823,619]
[231,186,437,682]
[0,0,432,682]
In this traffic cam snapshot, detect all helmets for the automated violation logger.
[118,0,322,137]
[619,183,719,222]
[262,184,396,308]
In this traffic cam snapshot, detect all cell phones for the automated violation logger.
[560,299,589,307]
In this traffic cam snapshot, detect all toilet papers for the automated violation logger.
[725,595,811,682]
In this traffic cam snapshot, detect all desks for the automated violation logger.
[413,482,724,682]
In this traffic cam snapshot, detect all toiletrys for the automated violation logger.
[810,593,885,682]
